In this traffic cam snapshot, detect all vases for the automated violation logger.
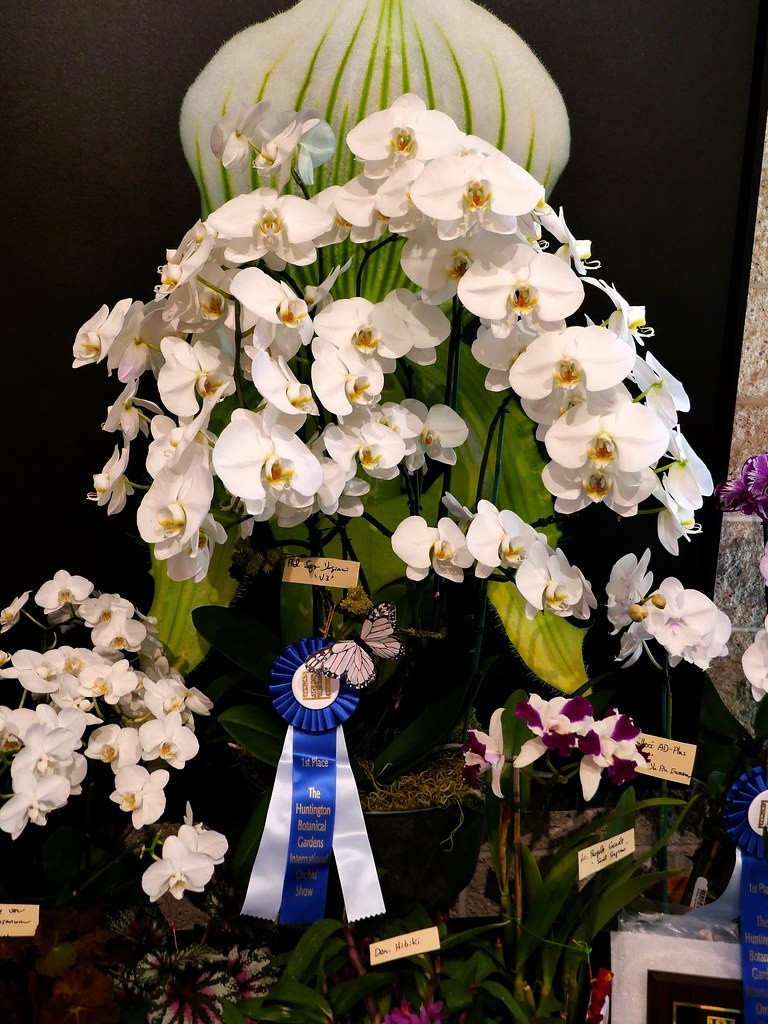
[327,805,482,932]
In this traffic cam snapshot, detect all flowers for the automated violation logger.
[0,84,767,1024]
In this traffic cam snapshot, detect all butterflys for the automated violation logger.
[305,603,406,690]
